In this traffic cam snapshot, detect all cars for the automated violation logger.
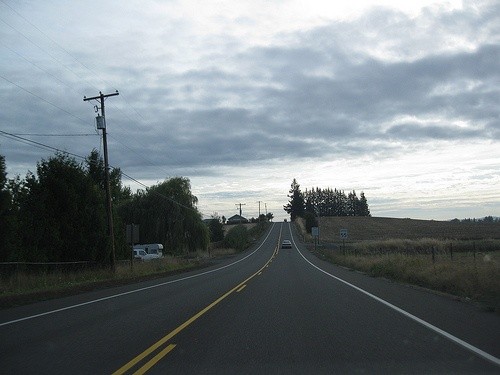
[142,253,158,260]
[282,240,292,249]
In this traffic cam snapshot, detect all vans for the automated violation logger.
[134,249,147,258]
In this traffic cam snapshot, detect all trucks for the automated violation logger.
[133,244,163,256]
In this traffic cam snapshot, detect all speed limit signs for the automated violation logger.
[340,231,348,240]
